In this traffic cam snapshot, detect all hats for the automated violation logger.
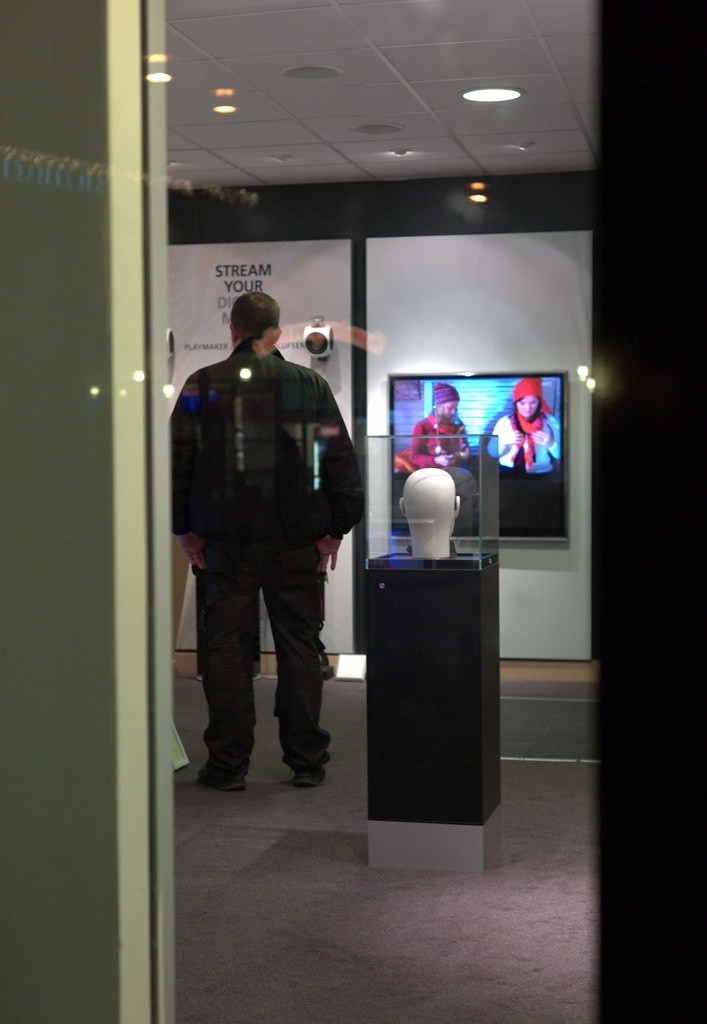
[513,378,543,402]
[434,383,460,405]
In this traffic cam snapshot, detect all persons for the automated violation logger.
[169,291,364,790]
[399,468,460,558]
[486,377,560,480]
[408,382,471,471]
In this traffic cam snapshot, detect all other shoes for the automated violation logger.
[292,768,325,786]
[199,767,246,791]
